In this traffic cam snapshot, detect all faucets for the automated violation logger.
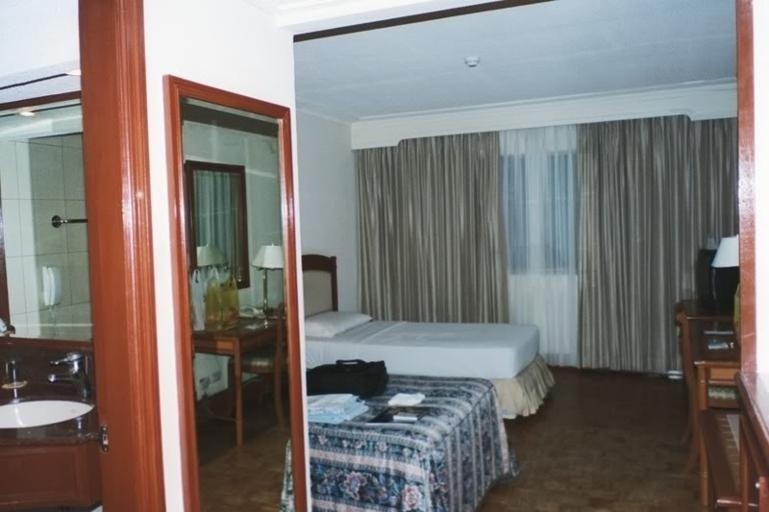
[48,351,91,399]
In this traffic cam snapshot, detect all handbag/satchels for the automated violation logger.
[306,356,390,398]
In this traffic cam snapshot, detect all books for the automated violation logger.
[365,405,430,426]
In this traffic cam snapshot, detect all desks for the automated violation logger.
[674,295,743,433]
[687,335,742,462]
[190,316,288,448]
[697,372,769,511]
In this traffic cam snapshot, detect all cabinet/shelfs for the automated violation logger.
[5,377,104,512]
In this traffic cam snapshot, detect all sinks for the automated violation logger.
[0,401,94,430]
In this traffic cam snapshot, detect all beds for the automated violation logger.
[300,253,554,419]
[306,369,520,512]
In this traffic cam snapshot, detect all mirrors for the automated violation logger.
[165,76,307,511]
[0,105,94,341]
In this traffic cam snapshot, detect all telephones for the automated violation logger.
[238,304,263,319]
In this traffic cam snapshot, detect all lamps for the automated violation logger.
[196,244,227,269]
[710,236,740,268]
[252,245,284,315]
